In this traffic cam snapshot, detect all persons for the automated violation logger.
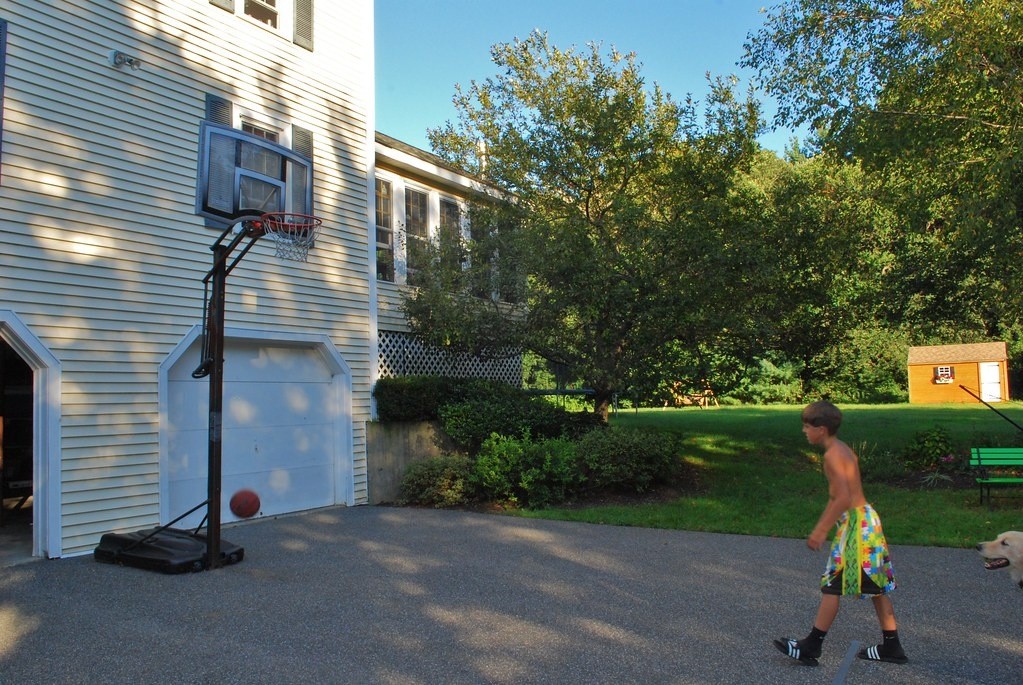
[772,400,907,667]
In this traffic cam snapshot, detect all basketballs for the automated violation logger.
[229,490,261,517]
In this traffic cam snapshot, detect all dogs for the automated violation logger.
[975,530,1023,588]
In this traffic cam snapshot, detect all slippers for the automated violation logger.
[857,644,908,663]
[772,638,819,666]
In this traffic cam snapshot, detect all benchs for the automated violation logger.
[970,446,1022,509]
